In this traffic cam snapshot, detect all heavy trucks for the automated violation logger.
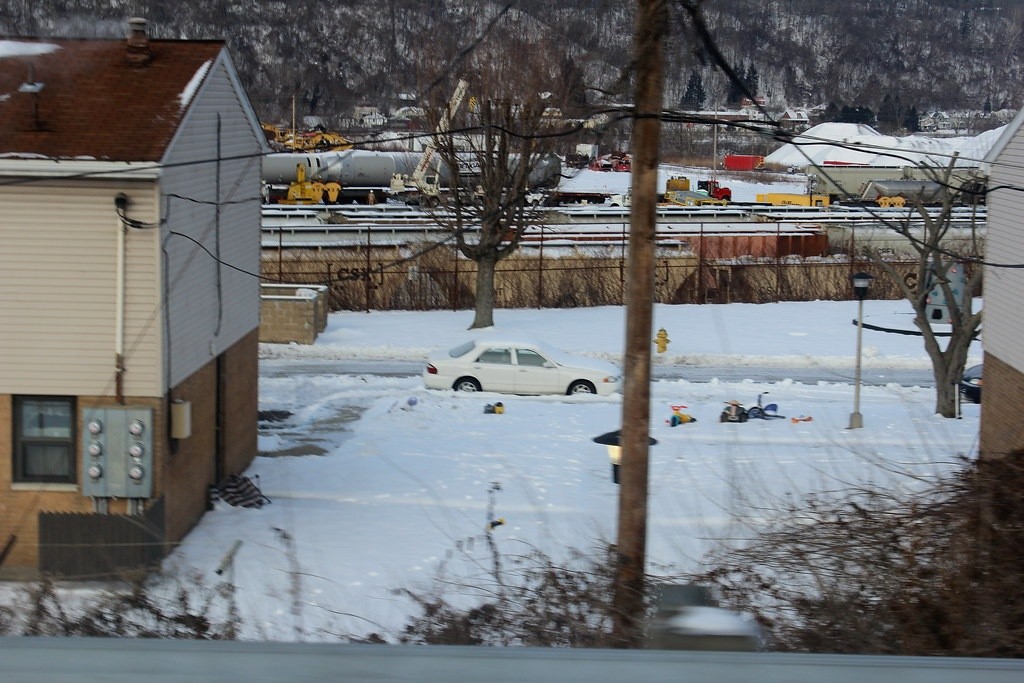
[627,177,740,207]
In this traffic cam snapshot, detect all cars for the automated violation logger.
[421,336,622,395]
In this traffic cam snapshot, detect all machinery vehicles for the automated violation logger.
[387,71,473,205]
[259,121,355,153]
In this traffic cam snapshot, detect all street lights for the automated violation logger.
[848,272,875,428]
